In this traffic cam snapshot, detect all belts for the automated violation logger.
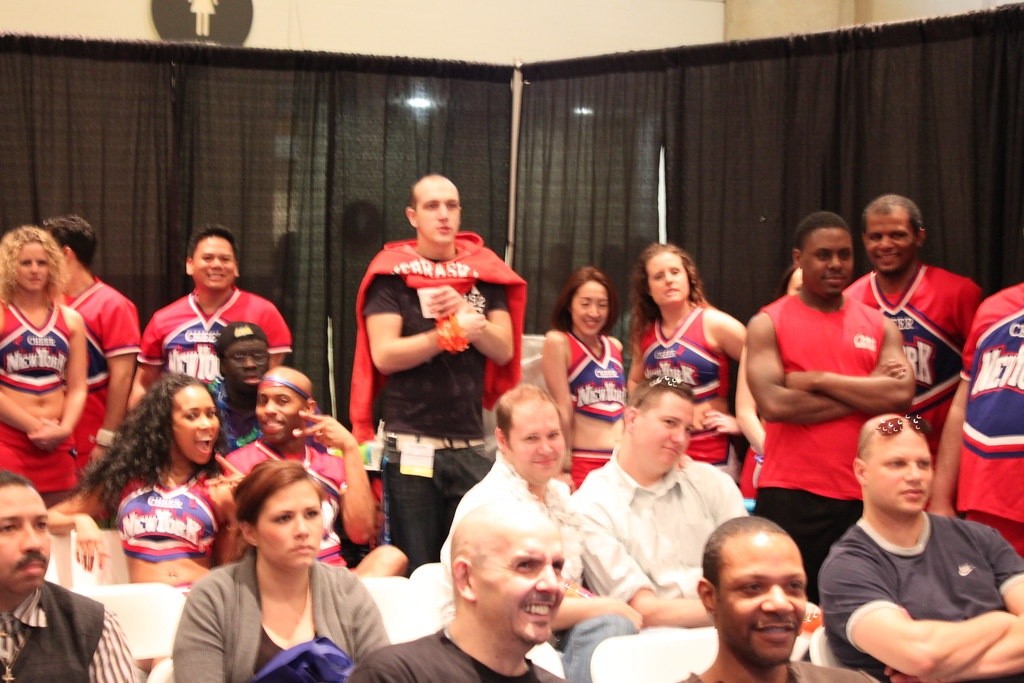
[384,430,486,454]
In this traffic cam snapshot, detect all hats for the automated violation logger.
[214,322,268,355]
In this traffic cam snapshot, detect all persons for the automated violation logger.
[96,428,115,447]
[627,243,747,488]
[926,281,1024,554]
[543,263,625,490]
[817,413,1024,683]
[364,173,513,579]
[346,382,643,683]
[841,195,984,467]
[0,212,394,683]
[325,201,384,430]
[736,208,916,605]
[572,371,881,683]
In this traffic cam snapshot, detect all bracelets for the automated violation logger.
[434,311,469,354]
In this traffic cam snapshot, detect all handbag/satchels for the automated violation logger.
[251,635,356,683]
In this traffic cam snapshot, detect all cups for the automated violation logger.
[417,288,440,318]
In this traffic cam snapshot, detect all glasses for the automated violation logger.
[634,376,692,406]
[858,414,926,455]
[222,350,271,368]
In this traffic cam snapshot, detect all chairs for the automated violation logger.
[43,529,848,683]
